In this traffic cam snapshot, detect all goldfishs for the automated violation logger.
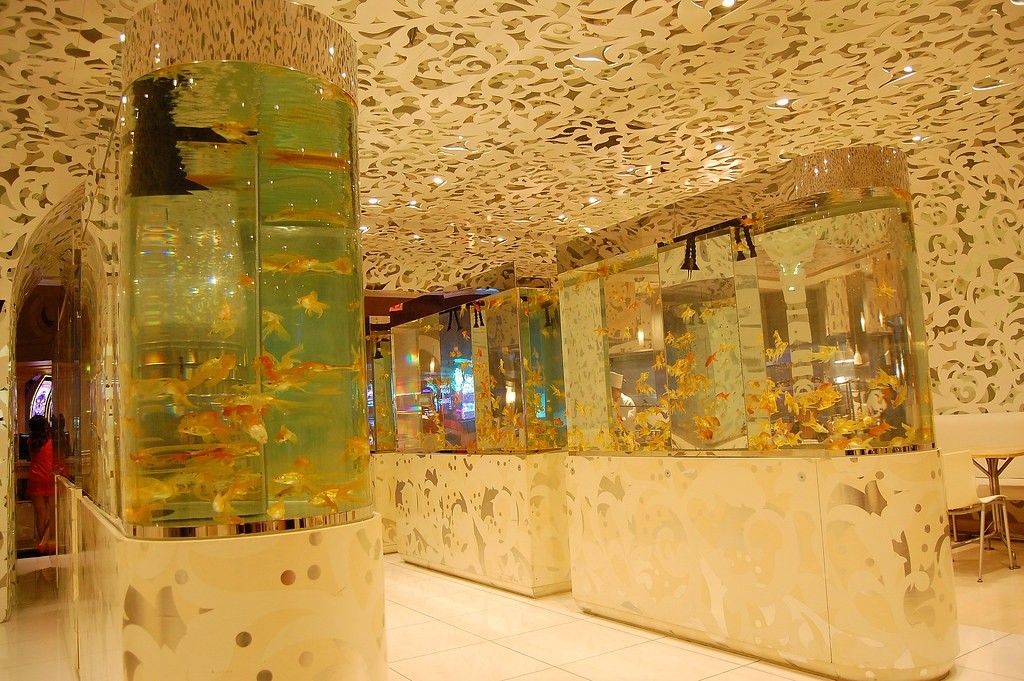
[740,188,917,451]
[118,83,367,527]
[367,287,592,450]
[594,235,735,441]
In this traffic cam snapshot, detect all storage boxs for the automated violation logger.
[364,315,394,559]
[16,500,39,542]
[557,147,956,681]
[388,260,573,598]
[49,0,389,681]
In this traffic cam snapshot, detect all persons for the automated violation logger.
[27,415,56,554]
[611,372,637,423]
[57,413,69,478]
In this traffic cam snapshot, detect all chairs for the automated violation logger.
[943,450,1013,583]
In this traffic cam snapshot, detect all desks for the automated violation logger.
[951,449,1024,569]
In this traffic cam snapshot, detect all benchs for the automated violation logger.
[934,412,1024,540]
